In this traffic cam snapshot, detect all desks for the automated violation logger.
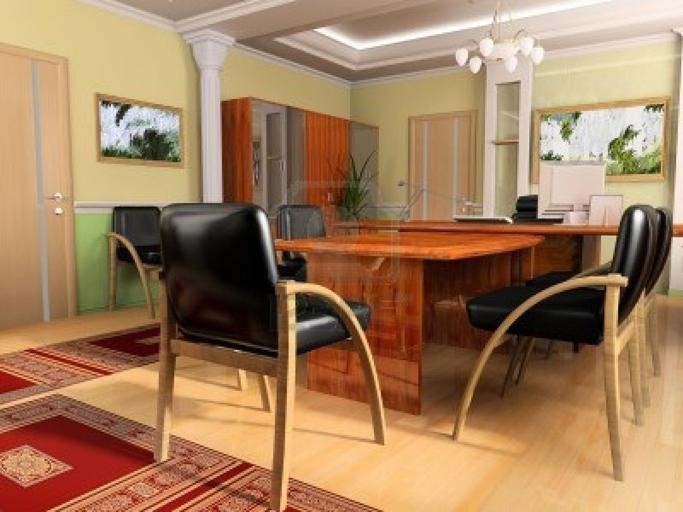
[272,222,683,417]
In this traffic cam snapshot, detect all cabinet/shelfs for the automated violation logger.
[221,98,380,236]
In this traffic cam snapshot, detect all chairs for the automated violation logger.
[451,203,673,481]
[511,196,562,224]
[107,206,163,321]
[153,204,388,512]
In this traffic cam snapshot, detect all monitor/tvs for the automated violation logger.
[537,158,608,225]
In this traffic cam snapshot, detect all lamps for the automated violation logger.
[396,178,490,219]
[456,0,545,78]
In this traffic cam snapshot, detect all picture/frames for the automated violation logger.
[530,96,673,185]
[588,195,623,228]
[94,94,185,168]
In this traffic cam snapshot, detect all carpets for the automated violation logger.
[0,322,160,404]
[1,394,381,511]
